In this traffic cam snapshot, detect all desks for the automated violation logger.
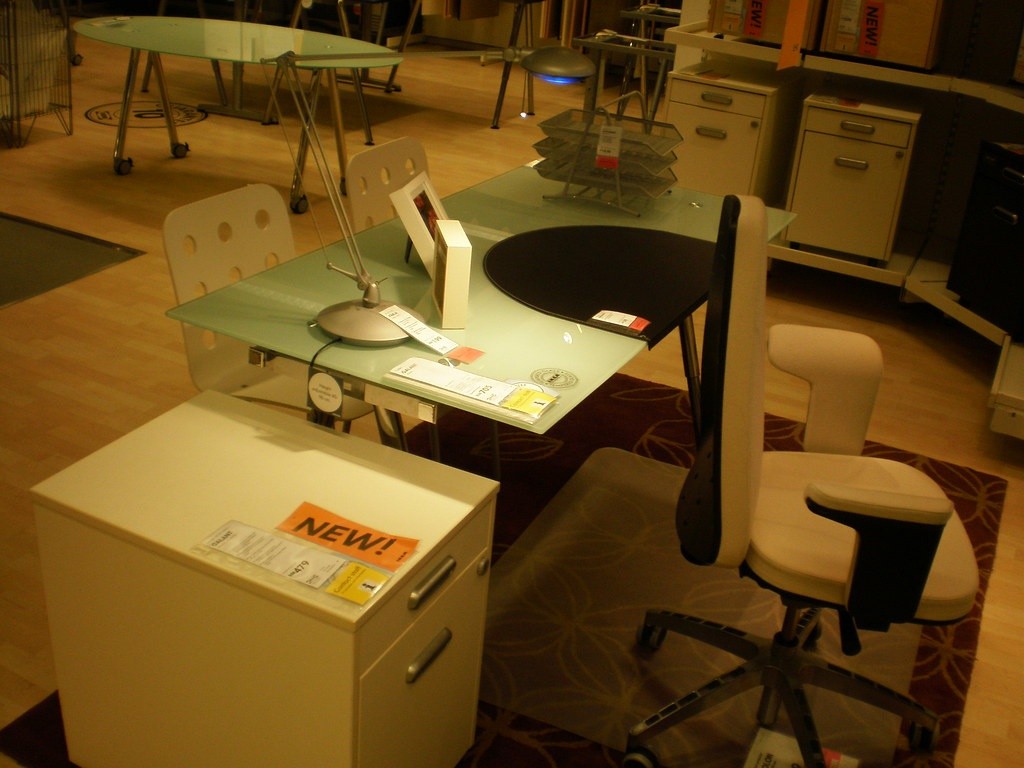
[72,12,404,218]
[166,163,797,552]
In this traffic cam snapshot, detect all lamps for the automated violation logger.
[257,43,597,348]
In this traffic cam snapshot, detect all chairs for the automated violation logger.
[169,182,409,462]
[342,133,430,235]
[618,192,983,768]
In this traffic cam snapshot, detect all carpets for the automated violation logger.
[0,374,1008,766]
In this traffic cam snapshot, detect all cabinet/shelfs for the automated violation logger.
[781,96,926,263]
[658,59,799,207]
[26,387,501,768]
